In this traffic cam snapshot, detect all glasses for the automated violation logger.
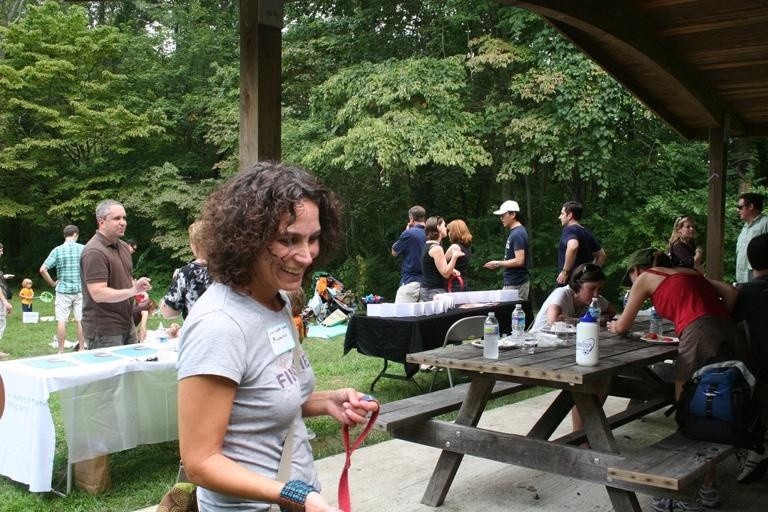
[737,204,745,210]
[582,264,602,274]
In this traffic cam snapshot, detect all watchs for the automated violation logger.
[562,270,567,275]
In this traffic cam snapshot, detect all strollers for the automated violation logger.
[311,270,360,325]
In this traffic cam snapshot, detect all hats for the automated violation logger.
[492,200,520,215]
[621,246,657,287]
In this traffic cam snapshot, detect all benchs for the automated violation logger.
[363,314,735,512]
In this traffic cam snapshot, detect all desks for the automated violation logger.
[343,296,533,394]
[0,336,182,498]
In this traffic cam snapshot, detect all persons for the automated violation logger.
[605,193,766,511]
[532,265,616,449]
[391,206,472,304]
[19,278,34,312]
[0,242,13,357]
[38,199,152,357]
[484,200,530,299]
[159,222,213,338]
[556,200,606,291]
[176,159,378,512]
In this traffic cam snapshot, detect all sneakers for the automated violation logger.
[695,487,721,508]
[736,442,768,483]
[648,496,704,512]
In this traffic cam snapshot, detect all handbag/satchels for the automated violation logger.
[155,465,197,512]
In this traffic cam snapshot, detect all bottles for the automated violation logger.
[649,308,662,336]
[483,304,526,359]
[155,321,168,355]
[575,297,602,367]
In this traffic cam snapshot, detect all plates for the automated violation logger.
[471,321,577,350]
[640,334,680,345]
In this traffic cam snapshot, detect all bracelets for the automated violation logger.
[278,479,319,512]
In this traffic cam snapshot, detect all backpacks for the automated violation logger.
[664,360,768,455]
